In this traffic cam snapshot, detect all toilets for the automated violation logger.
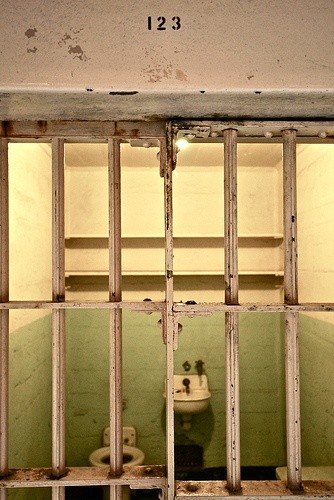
[87,424,145,500]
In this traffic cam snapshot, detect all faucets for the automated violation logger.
[195,359,204,376]
[182,360,192,372]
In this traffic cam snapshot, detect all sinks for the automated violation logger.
[162,372,212,415]
